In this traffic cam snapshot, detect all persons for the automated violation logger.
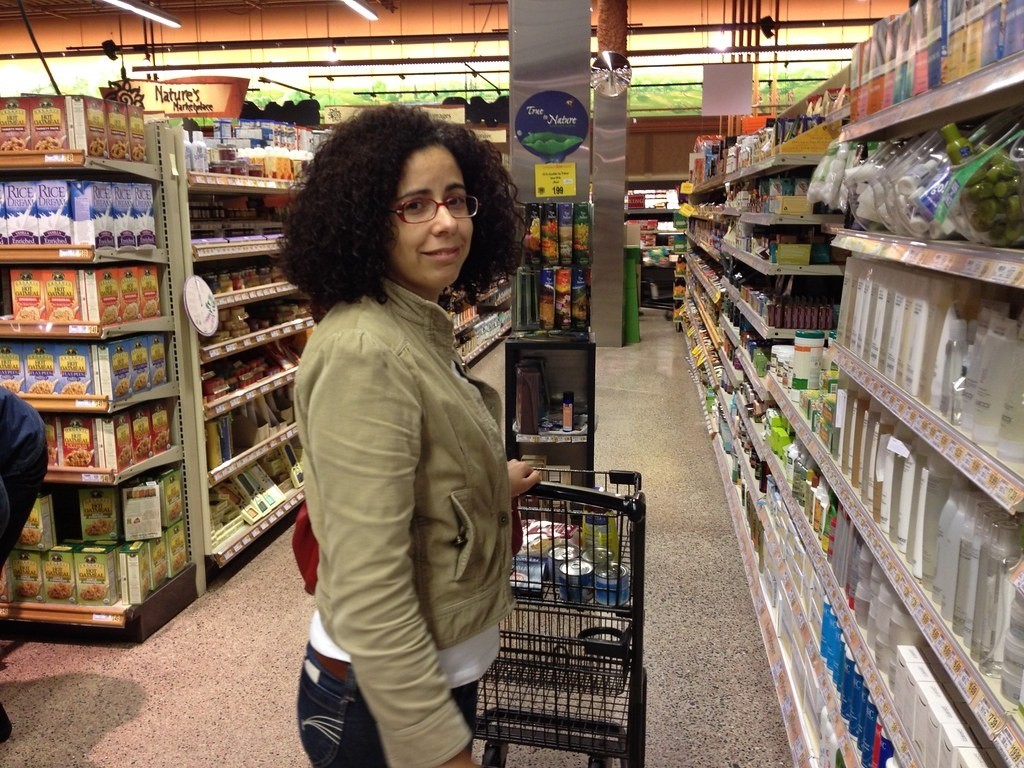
[280,106,541,768]
[0,384,49,743]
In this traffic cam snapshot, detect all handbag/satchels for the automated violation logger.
[291,497,523,597]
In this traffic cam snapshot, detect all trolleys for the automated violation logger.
[472,470,647,768]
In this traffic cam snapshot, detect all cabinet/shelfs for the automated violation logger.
[0,121,513,645]
[625,51,1024,768]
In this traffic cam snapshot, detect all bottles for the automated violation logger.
[189,201,290,240]
[185,119,330,180]
[846,103,1024,247]
[723,251,1024,767]
[582,483,621,565]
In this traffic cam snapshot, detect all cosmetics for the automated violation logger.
[833,258,1024,466]
[819,501,1008,768]
[843,119,1024,249]
[826,370,1024,715]
[713,270,847,574]
[760,408,835,557]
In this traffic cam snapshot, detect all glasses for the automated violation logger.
[389,195,482,224]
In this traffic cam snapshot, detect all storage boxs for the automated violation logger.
[0,95,190,612]
